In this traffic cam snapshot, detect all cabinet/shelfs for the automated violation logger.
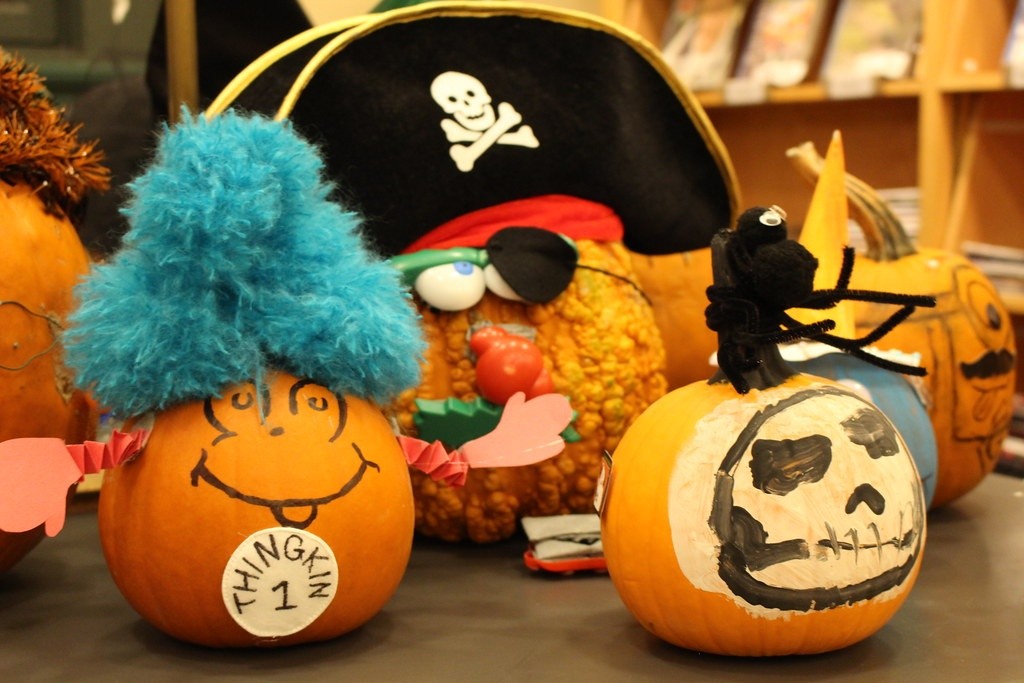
[627,0,1024,248]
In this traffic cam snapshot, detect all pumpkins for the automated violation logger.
[0,129,1016,659]
